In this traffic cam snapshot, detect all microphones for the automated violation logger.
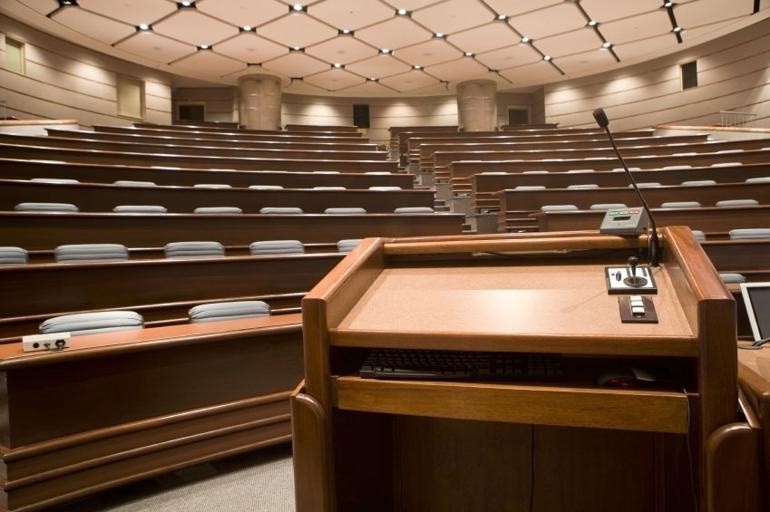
[593,108,660,265]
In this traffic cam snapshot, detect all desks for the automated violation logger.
[0,123,770,512]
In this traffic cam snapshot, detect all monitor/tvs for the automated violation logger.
[739,281,770,342]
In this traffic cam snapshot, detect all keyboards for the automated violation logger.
[360,351,556,382]
